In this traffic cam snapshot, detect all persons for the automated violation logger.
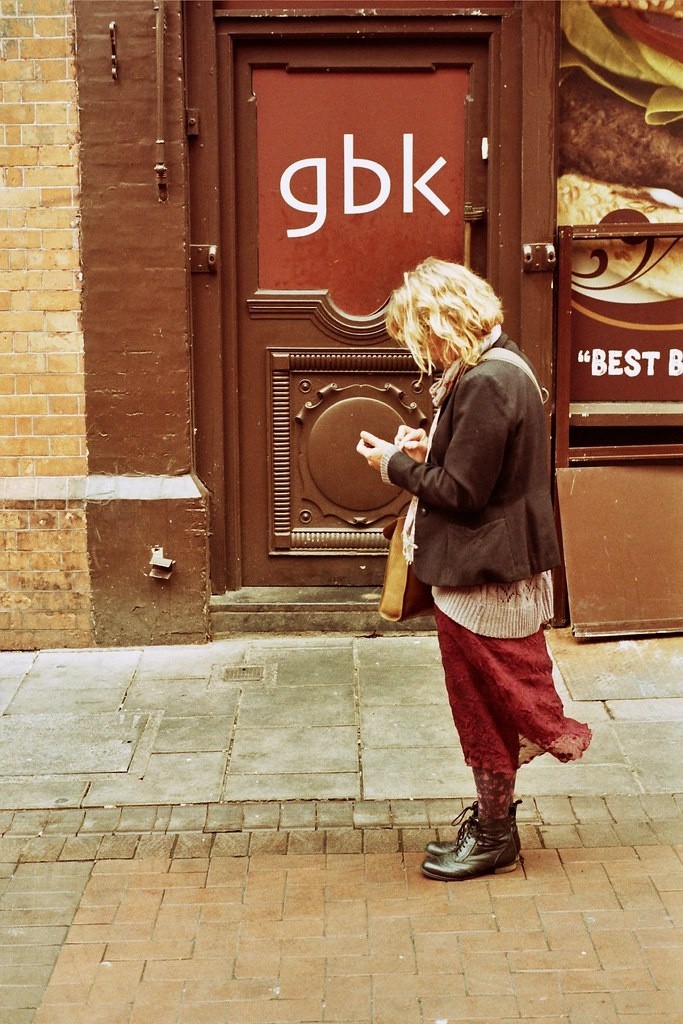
[354,256,592,881]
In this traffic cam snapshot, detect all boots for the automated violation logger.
[421,815,516,880]
[425,799,521,861]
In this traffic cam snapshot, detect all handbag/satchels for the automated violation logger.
[377,515,434,622]
